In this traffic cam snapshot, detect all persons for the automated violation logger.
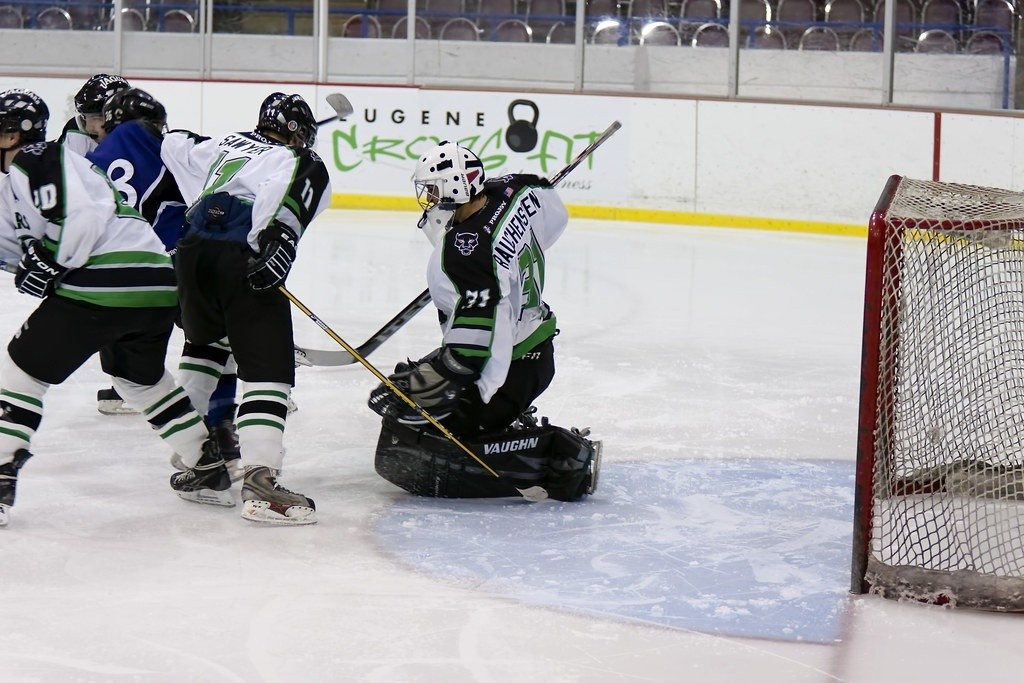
[0,74,331,527]
[368,138,604,502]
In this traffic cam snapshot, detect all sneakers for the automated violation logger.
[0,448,36,525]
[168,440,237,507]
[240,466,318,524]
[170,402,245,482]
[96,385,141,413]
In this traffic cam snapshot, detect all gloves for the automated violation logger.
[245,221,299,292]
[13,240,67,298]
[387,353,481,412]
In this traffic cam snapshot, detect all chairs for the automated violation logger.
[0,0,1020,52]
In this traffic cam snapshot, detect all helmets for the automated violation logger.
[257,92,317,148]
[103,89,169,132]
[410,142,486,228]
[75,74,133,116]
[0,89,50,149]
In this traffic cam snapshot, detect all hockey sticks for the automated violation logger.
[291,120,624,369]
[247,254,550,504]
